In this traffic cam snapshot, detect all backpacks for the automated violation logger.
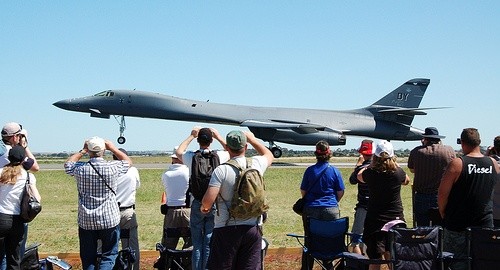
[215,157,269,226]
[189,150,220,202]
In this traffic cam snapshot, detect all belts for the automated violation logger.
[167,206,190,209]
[119,206,132,209]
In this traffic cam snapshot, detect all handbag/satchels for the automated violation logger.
[20,184,42,223]
[293,199,303,216]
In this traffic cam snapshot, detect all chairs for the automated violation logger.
[448,224,499,270]
[285,217,365,269]
[155,243,194,270]
[372,226,455,270]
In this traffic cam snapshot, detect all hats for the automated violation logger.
[357,140,373,155]
[8,145,25,164]
[87,137,106,151]
[16,129,28,143]
[375,140,394,158]
[198,128,212,143]
[226,130,247,149]
[422,127,446,138]
[169,146,186,159]
[0,122,22,136]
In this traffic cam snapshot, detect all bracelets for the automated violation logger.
[79,150,85,156]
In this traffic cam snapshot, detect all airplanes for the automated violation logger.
[52,78,453,158]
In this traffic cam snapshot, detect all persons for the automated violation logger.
[112,149,140,270]
[438,127,500,270]
[408,127,456,226]
[200,129,274,270]
[356,140,410,269]
[159,146,193,250]
[485,136,500,228]
[175,126,231,270]
[300,140,346,270]
[64,136,132,270]
[0,122,40,270]
[348,139,377,255]
[0,143,36,270]
